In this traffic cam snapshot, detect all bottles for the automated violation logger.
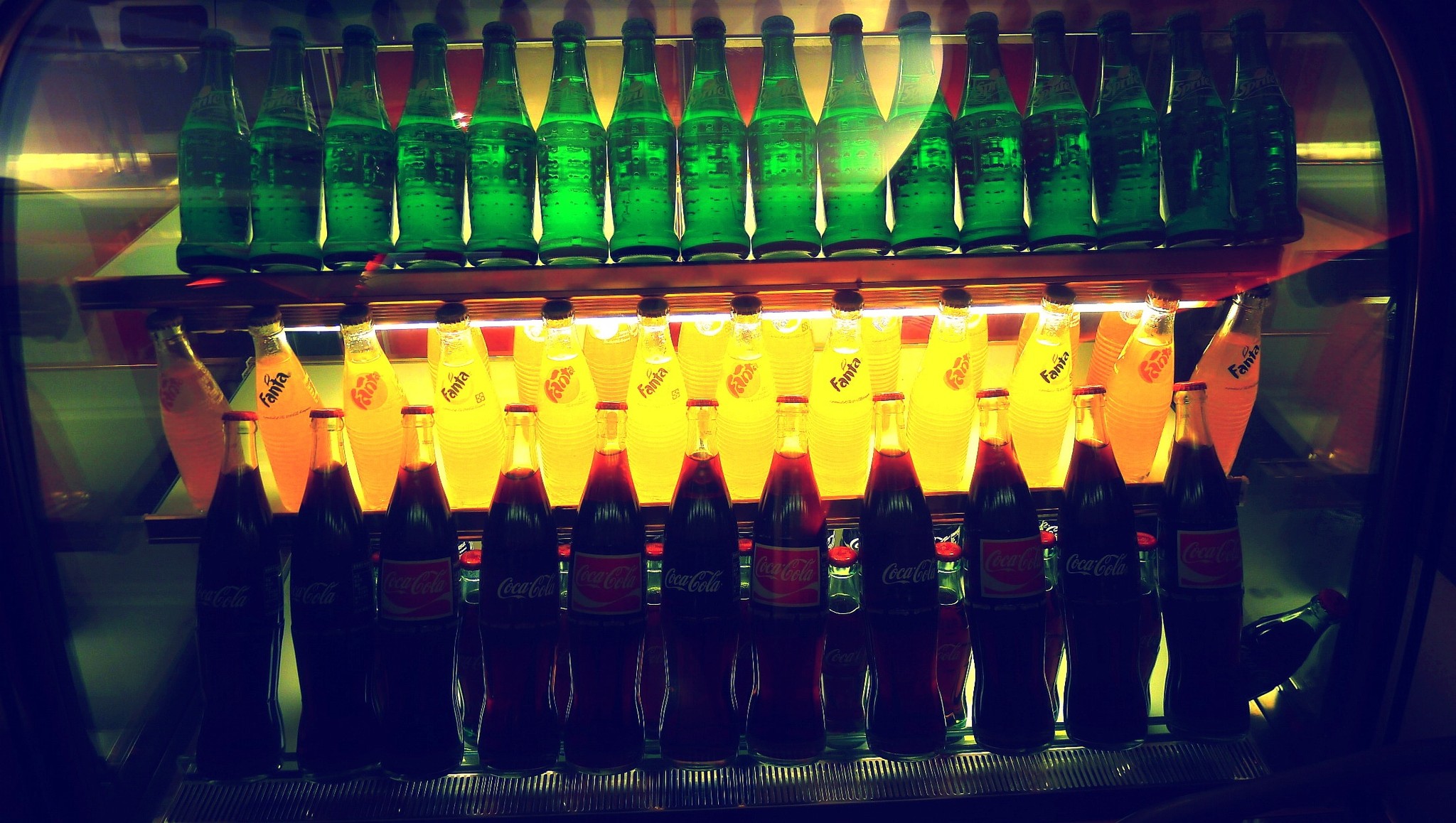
[250,24,323,272]
[606,17,681,263]
[953,9,1030,255]
[464,21,538,266]
[1087,10,1167,250]
[176,28,253,275]
[816,12,892,258]
[746,16,821,259]
[535,18,608,266]
[1022,10,1102,252]
[677,17,750,261]
[394,23,465,269]
[1226,8,1305,247]
[320,24,395,270]
[141,280,1270,785]
[1302,298,1389,474]
[885,11,960,256]
[1242,589,1353,700]
[1157,10,1239,249]
